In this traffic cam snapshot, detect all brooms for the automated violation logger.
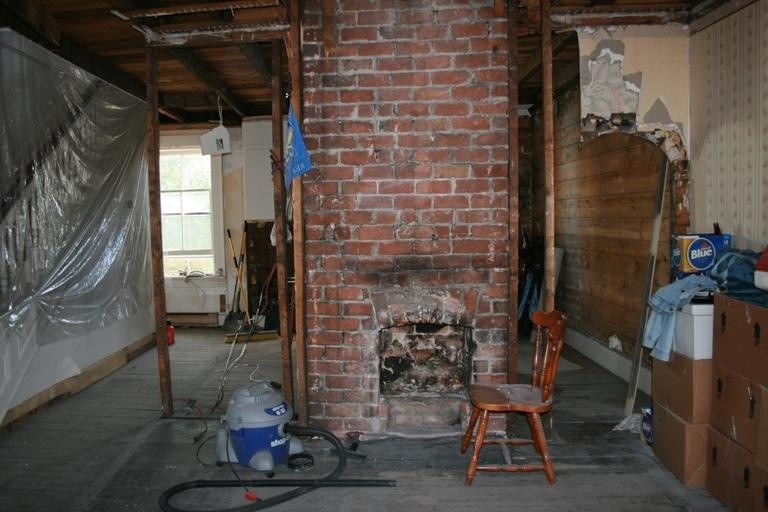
[224,229,279,345]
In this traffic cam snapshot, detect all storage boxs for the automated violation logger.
[651,232,768,512]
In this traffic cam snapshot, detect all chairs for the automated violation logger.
[460,310,566,485]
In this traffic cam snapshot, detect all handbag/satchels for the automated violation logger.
[711,248,758,285]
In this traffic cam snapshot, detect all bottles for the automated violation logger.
[165,320,175,345]
